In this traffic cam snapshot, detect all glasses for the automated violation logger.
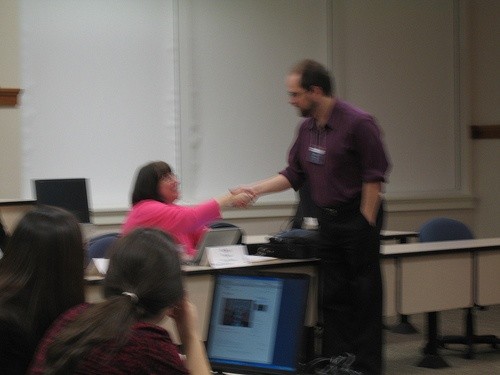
[287,87,314,100]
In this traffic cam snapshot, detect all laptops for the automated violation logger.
[184,227,244,264]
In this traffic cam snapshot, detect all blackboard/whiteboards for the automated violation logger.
[0,0,475,211]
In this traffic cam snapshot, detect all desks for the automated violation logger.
[81,228,500,370]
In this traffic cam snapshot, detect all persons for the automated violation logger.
[229,61,388,375]
[0,205,85,375]
[29,229,212,375]
[121,162,255,255]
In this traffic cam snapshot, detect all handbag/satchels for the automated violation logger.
[245,228,320,260]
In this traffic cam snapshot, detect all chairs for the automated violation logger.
[83,236,118,268]
[205,223,242,247]
[416,217,500,360]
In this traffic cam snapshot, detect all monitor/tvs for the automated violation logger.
[35,178,90,223]
[205,272,312,375]
